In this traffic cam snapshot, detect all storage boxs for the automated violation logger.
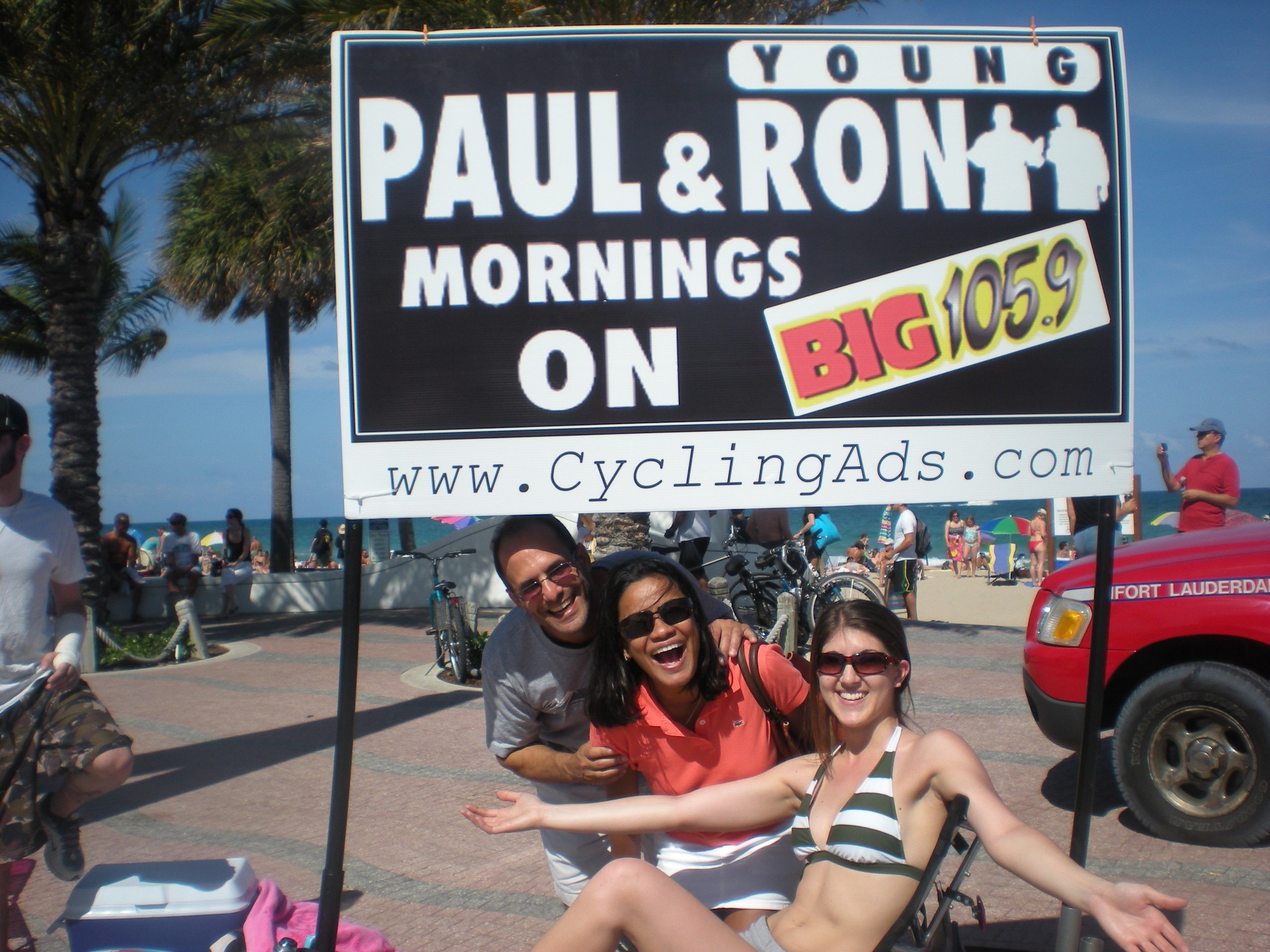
[50,857,260,952]
[1055,557,1073,571]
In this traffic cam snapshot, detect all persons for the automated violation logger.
[1056,541,1070,558]
[102,512,147,623]
[1157,418,1240,533]
[212,508,251,621]
[102,507,270,578]
[0,393,134,882]
[460,514,1191,952]
[664,507,829,592]
[293,519,397,572]
[161,513,202,622]
[1067,495,1137,560]
[1027,508,1049,587]
[890,504,919,620]
[833,509,991,587]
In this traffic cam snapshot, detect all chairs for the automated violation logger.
[824,556,850,576]
[987,543,1016,585]
[872,792,986,952]
[885,562,918,617]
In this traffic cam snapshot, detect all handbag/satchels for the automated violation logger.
[809,512,842,551]
[210,561,222,577]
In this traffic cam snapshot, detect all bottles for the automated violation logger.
[272,937,297,952]
[1124,494,1134,514]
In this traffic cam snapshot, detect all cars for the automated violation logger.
[1020,516,1270,849]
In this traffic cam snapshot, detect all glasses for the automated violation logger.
[952,515,959,518]
[616,598,698,640]
[224,515,237,521]
[814,652,900,675]
[509,549,576,601]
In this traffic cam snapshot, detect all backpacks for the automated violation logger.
[913,513,932,558]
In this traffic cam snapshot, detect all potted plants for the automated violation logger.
[467,631,491,678]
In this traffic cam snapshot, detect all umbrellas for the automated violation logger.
[126,528,147,547]
[974,514,1031,545]
[200,529,223,547]
[431,516,483,531]
[1151,511,1181,529]
[877,504,892,547]
[1225,508,1262,526]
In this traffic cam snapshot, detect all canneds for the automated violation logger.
[1124,493,1134,514]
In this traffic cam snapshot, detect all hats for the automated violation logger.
[0,394,28,433]
[338,524,345,534]
[1037,508,1047,518]
[165,513,186,523]
[320,518,327,526]
[1189,418,1227,439]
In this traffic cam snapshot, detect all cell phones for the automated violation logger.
[1161,443,1168,451]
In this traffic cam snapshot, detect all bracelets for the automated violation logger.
[799,531,802,535]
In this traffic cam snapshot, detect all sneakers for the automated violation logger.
[35,792,85,882]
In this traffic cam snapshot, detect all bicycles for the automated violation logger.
[390,547,482,683]
[645,513,889,653]
